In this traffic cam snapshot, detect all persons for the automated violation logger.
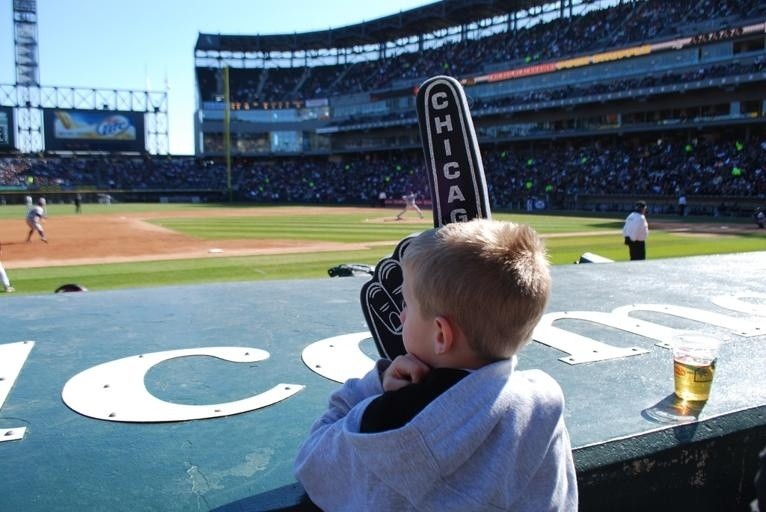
[200,2,766,220]
[753,207,766,228]
[25,196,49,244]
[0,148,199,216]
[396,190,426,222]
[622,199,649,260]
[0,247,16,294]
[293,217,581,512]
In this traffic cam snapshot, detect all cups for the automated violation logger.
[640,391,698,424]
[672,331,721,402]
